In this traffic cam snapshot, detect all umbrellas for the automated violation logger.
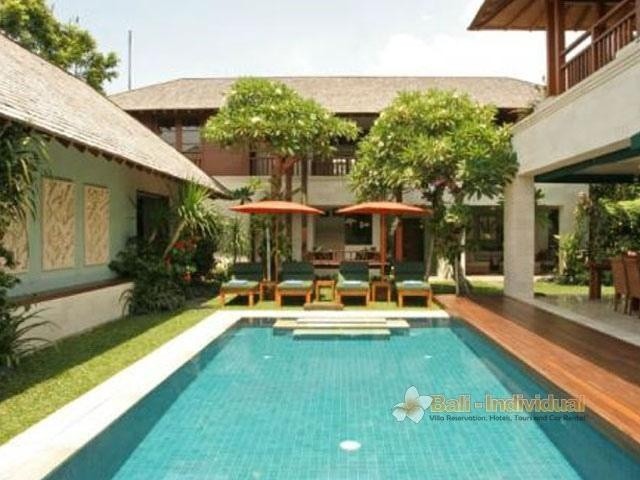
[229,199,327,281]
[334,199,430,273]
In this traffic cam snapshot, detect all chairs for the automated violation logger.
[609,256,640,318]
[220,258,431,310]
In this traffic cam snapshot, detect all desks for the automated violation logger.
[591,261,612,299]
